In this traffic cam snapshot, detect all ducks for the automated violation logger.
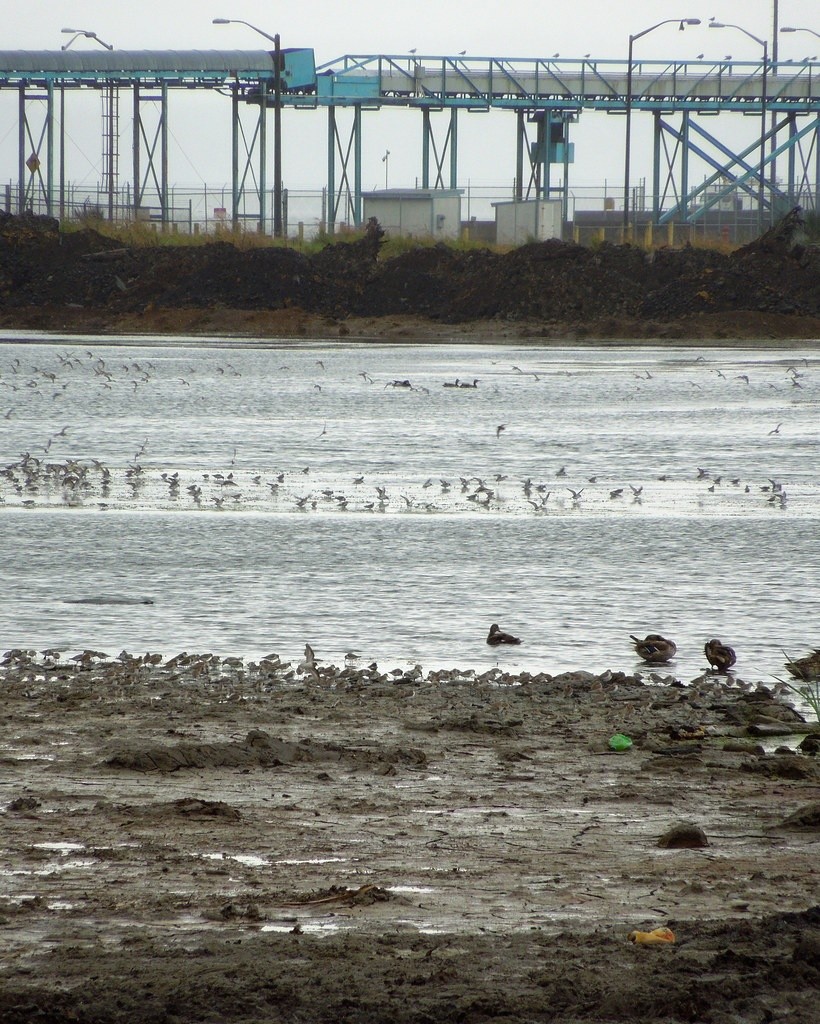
[392,379,411,387]
[442,378,483,389]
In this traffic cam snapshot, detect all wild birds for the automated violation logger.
[409,385,429,395]
[703,639,737,671]
[488,354,816,394]
[0,643,793,704]
[487,624,524,646]
[629,634,677,662]
[0,421,814,511]
[783,648,820,682]
[0,347,395,420]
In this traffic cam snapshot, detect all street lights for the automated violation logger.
[709,22,769,237]
[59,25,114,221]
[624,16,701,253]
[780,27,820,221]
[210,18,288,240]
